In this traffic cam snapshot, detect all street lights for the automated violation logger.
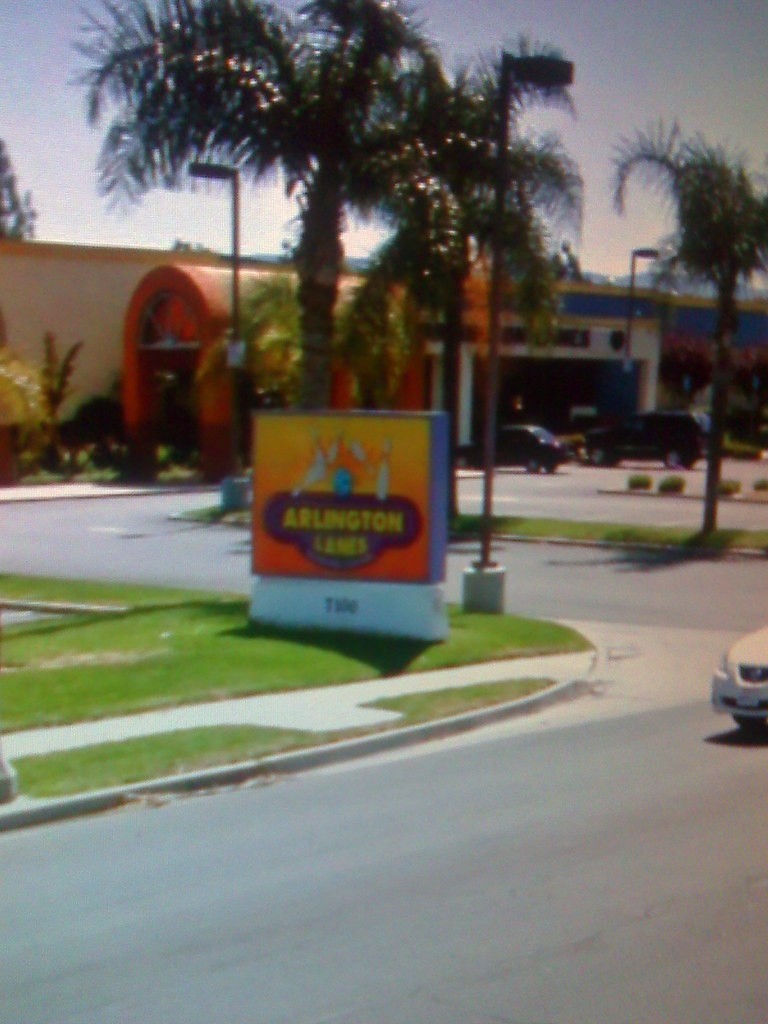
[188,162,250,514]
[601,248,658,467]
[463,53,572,606]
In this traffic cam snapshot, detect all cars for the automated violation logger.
[454,425,569,474]
[711,627,768,730]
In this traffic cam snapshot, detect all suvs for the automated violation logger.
[574,408,709,471]
